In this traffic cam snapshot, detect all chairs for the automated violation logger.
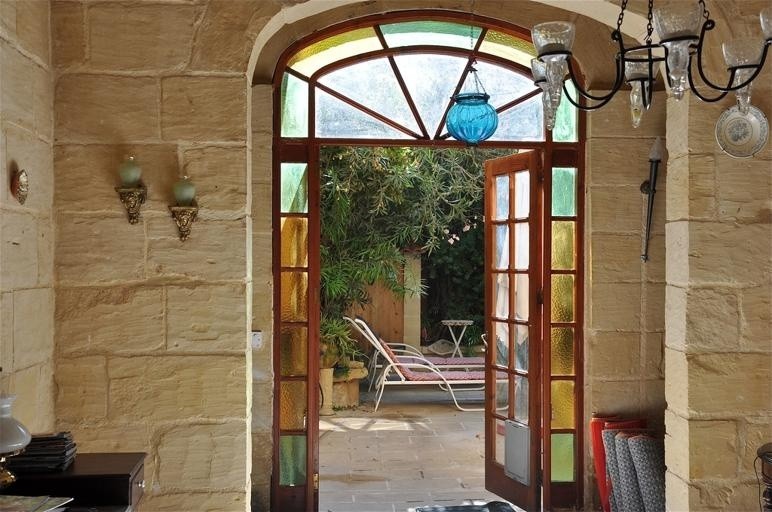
[342,315,529,412]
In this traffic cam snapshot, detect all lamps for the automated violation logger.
[112,153,201,245]
[529,3,772,130]
[0,393,34,487]
[446,1,500,146]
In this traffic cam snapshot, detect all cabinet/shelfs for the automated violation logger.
[0,451,150,512]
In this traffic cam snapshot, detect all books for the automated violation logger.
[0,430,79,512]
